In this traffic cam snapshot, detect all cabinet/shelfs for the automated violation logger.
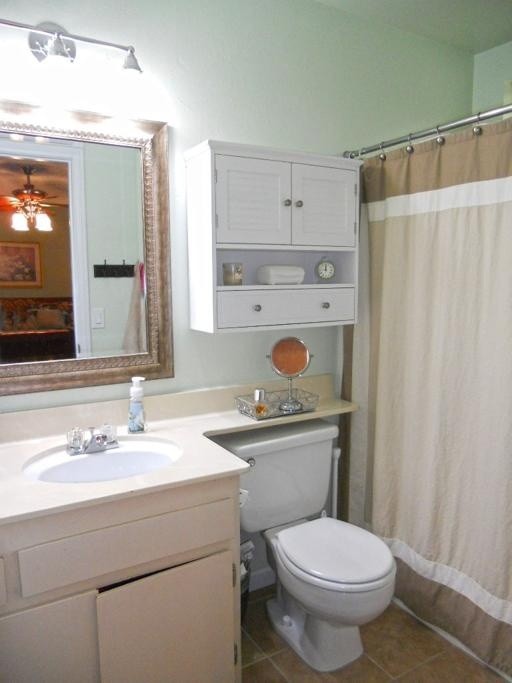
[0,476,241,682]
[187,140,364,333]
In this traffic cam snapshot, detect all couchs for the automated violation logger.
[0,298,74,353]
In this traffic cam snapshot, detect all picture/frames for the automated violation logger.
[0,241,42,288]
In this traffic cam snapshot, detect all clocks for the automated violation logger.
[315,256,336,279]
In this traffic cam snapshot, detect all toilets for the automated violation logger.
[216,418,397,672]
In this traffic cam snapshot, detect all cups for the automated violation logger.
[223,263,243,285]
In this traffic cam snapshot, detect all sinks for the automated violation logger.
[20,436,184,484]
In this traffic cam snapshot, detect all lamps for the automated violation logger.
[11,206,54,231]
[0,18,140,72]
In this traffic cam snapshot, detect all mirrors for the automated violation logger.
[0,100,175,395]
[266,336,314,413]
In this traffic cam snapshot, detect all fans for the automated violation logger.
[0,166,68,215]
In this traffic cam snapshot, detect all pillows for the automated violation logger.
[15,303,72,329]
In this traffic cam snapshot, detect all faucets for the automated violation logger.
[84,427,108,454]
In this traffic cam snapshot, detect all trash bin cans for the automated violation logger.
[241,567,250,626]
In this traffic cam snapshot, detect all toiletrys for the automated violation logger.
[253,387,269,415]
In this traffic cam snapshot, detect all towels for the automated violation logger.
[123,263,146,353]
